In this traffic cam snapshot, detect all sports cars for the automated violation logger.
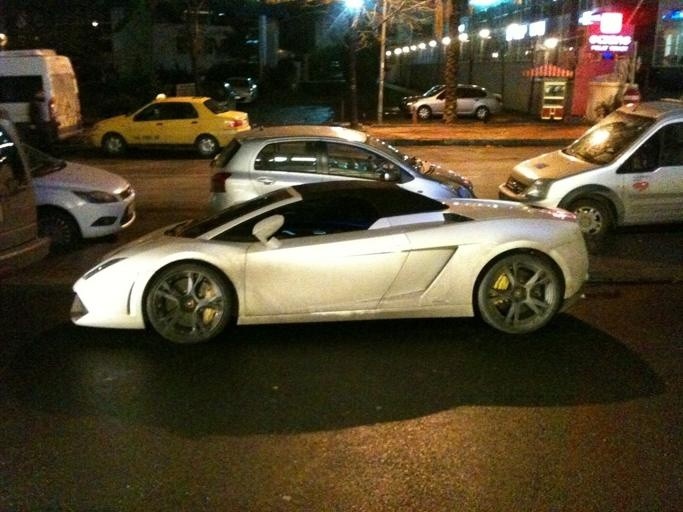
[51,174,598,350]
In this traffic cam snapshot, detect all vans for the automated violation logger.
[0,49,88,154]
[0,114,61,279]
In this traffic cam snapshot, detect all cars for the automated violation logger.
[21,143,138,246]
[402,83,504,125]
[495,96,683,244]
[91,92,252,158]
[210,125,478,208]
[222,76,259,104]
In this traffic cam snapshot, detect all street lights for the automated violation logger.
[343,0,362,128]
[88,16,102,87]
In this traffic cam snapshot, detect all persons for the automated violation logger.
[25,84,55,153]
